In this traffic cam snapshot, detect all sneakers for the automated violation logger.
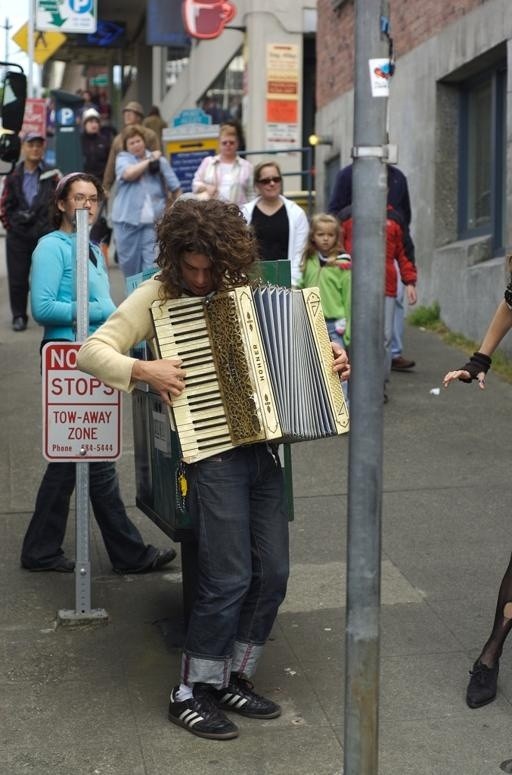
[13,312,28,331]
[392,356,415,368]
[167,683,240,739]
[211,676,282,720]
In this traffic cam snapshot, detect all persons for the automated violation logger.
[21,170,178,575]
[77,199,352,740]
[442,282,510,708]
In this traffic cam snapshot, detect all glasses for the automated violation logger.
[64,196,101,206]
[256,176,281,185]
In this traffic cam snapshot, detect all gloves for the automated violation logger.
[455,352,492,383]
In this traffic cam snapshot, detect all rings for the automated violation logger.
[452,370,457,373]
[478,379,485,383]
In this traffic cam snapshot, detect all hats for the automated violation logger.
[22,131,45,142]
[81,108,101,123]
[123,101,145,117]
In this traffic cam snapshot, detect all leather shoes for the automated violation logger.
[465,655,500,709]
[54,558,76,572]
[158,548,177,565]
